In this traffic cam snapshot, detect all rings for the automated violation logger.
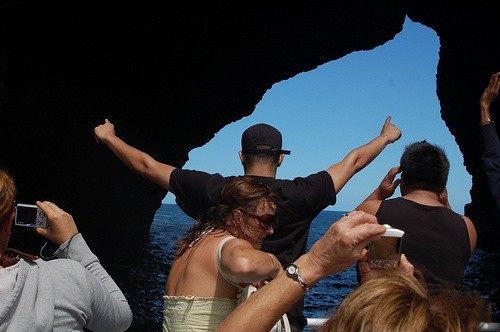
[345,214,350,218]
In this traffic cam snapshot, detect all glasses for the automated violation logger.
[245,211,276,231]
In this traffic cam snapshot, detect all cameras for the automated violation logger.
[13,203,49,228]
[366,223,405,271]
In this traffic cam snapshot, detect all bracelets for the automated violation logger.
[268,253,275,275]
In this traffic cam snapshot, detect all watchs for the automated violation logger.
[483,117,493,125]
[287,262,312,293]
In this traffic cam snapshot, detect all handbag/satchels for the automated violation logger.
[215,234,291,331]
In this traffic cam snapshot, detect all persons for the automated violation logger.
[94,115,401,332]
[479,72,500,212]
[353,138,477,288]
[162,175,284,332]
[213,209,492,332]
[0,167,133,332]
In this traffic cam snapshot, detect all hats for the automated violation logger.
[241,123,291,157]
